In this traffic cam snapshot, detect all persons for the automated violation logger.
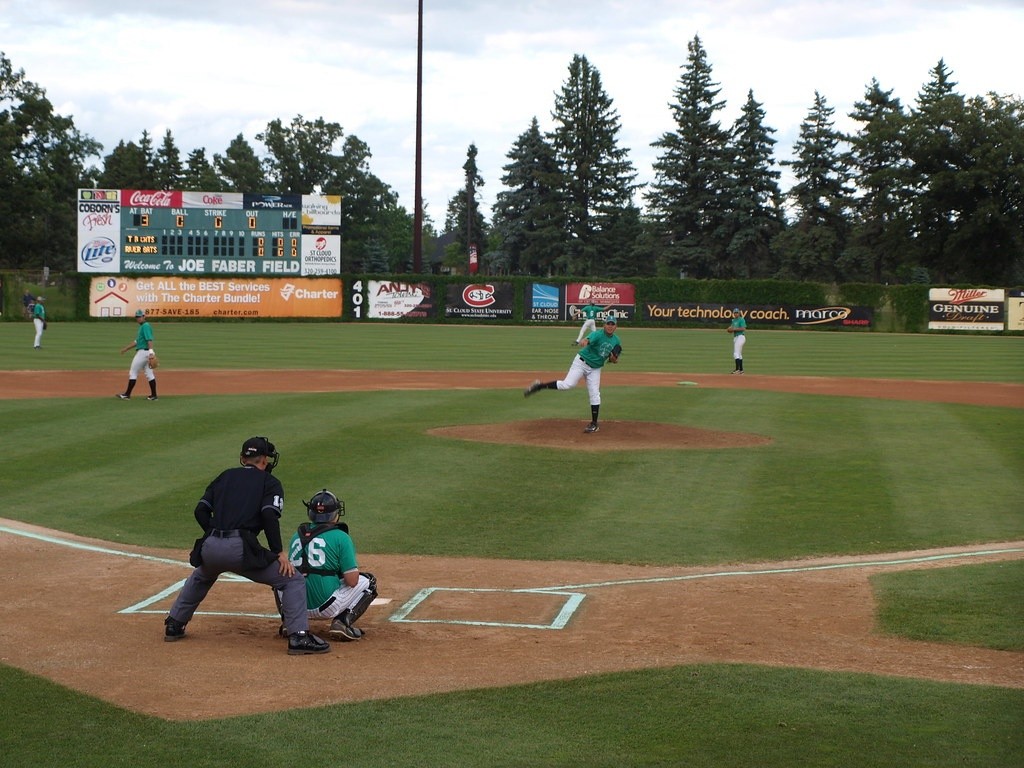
[22,289,47,350]
[270,488,379,641]
[728,308,747,374]
[164,434,331,655]
[572,300,616,346]
[524,316,623,432]
[115,308,159,401]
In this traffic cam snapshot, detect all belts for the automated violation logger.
[138,349,149,351]
[213,529,253,537]
[319,597,336,612]
[579,356,593,368]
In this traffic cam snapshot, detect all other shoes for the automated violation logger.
[33,345,43,350]
[732,369,744,375]
[574,341,578,344]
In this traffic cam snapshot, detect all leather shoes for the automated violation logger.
[287,632,330,654]
[164,616,185,640]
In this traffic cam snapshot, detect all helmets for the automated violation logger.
[308,491,340,522]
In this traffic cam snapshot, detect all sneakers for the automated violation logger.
[585,424,600,433]
[328,619,363,640]
[115,393,130,401]
[142,396,158,401]
[525,379,539,398]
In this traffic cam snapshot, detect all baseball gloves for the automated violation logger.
[148,355,159,369]
[728,327,734,333]
[609,345,621,363]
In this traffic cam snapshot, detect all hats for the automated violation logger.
[37,296,46,301]
[242,437,276,455]
[135,310,144,316]
[733,308,739,313]
[606,316,616,325]
